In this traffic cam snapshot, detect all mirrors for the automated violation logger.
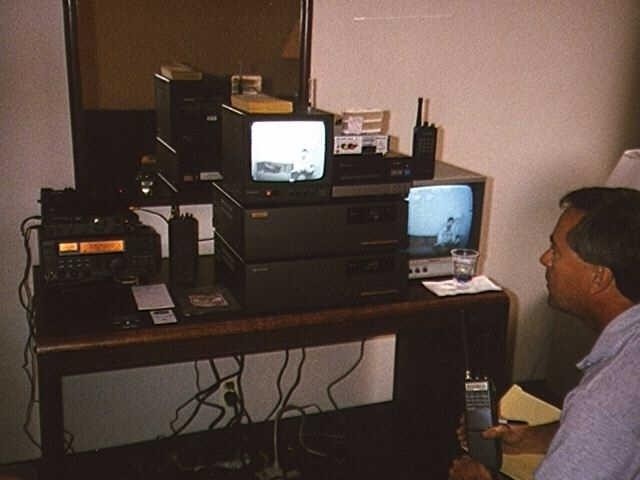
[63,2,313,210]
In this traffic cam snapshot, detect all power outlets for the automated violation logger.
[219,378,238,407]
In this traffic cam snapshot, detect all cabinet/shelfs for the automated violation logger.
[151,71,225,199]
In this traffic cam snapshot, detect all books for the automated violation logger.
[160,63,202,80]
[230,94,293,114]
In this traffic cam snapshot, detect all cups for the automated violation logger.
[450,247,480,289]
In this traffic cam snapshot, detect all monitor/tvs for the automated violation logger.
[154,71,228,154]
[221,103,335,205]
[405,160,494,281]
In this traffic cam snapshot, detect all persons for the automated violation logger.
[436,217,461,247]
[448,186,640,480]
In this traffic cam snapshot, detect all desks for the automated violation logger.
[31,263,519,478]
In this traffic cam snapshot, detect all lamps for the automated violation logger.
[605,148,638,188]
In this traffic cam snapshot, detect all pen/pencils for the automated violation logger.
[498,419,528,425]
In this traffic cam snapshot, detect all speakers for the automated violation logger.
[168,216,198,291]
